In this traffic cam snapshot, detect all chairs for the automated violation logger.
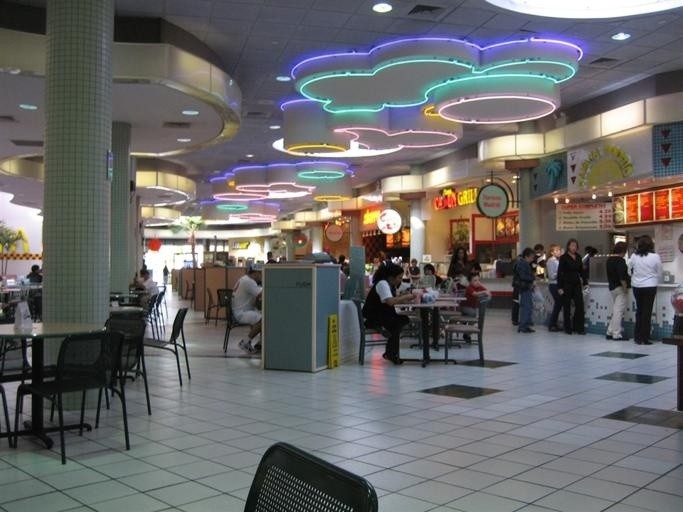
[94,317,151,429]
[157,286,168,317]
[183,280,193,299]
[449,292,488,345]
[153,292,165,335]
[222,292,253,354]
[243,440,378,512]
[13,331,130,465]
[17,337,58,422]
[145,294,158,339]
[442,298,486,367]
[138,308,190,387]
[351,298,396,366]
[129,284,137,291]
[215,288,234,326]
[204,288,218,326]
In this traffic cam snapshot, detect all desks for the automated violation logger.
[660,335,682,412]
[110,299,139,305]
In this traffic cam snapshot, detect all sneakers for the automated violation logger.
[254,343,261,350]
[549,325,563,331]
[238,339,256,353]
[641,339,652,344]
[565,328,571,333]
[606,334,612,339]
[613,335,628,340]
[578,330,585,334]
[518,327,535,332]
[383,353,402,364]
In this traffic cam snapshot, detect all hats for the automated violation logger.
[250,263,263,271]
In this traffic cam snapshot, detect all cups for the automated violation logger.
[412,289,424,304]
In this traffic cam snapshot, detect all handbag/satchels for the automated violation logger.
[512,273,528,289]
[531,286,544,302]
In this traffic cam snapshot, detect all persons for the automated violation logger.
[606,241,631,340]
[423,264,443,289]
[230,263,262,354]
[323,246,337,263]
[512,247,536,333]
[448,247,476,282]
[134,270,159,306]
[582,246,598,277]
[373,251,401,298]
[361,264,418,365]
[459,271,492,343]
[405,259,421,279]
[266,252,277,263]
[530,244,547,279]
[511,255,523,325]
[338,256,347,270]
[627,236,664,345]
[27,265,42,283]
[556,239,591,335]
[546,244,562,331]
